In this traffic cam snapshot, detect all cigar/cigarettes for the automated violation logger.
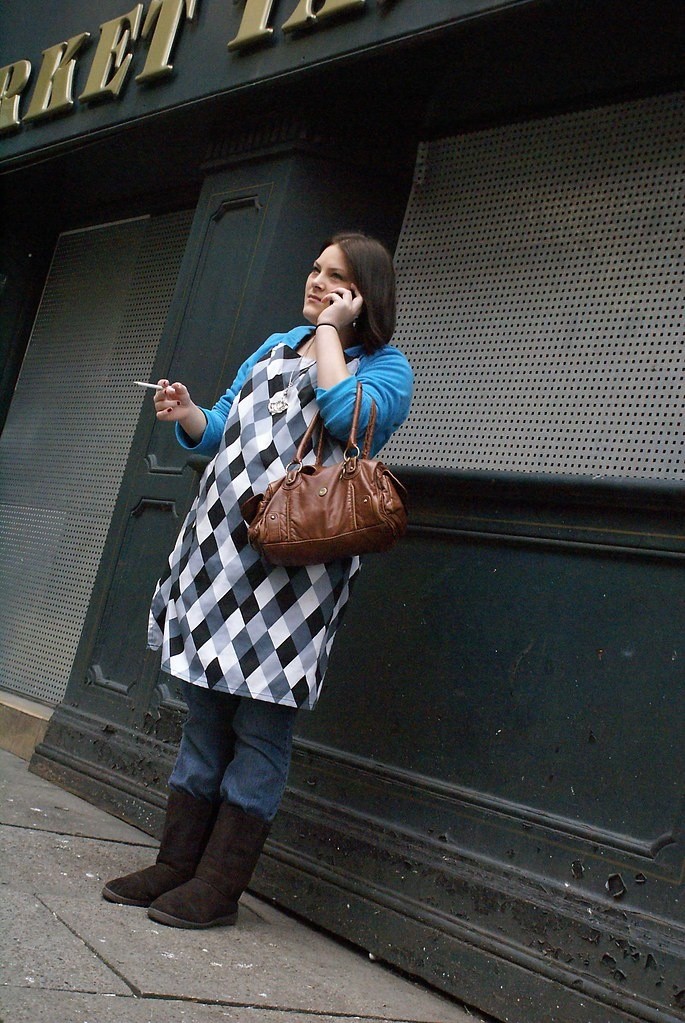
[134,382,163,389]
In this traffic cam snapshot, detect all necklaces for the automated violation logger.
[268,336,317,416]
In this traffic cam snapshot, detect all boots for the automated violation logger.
[147,802,273,929]
[102,791,224,907]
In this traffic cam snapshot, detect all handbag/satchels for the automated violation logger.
[240,381,408,568]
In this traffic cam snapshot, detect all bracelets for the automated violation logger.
[315,323,338,331]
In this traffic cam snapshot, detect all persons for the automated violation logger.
[101,232,414,929]
